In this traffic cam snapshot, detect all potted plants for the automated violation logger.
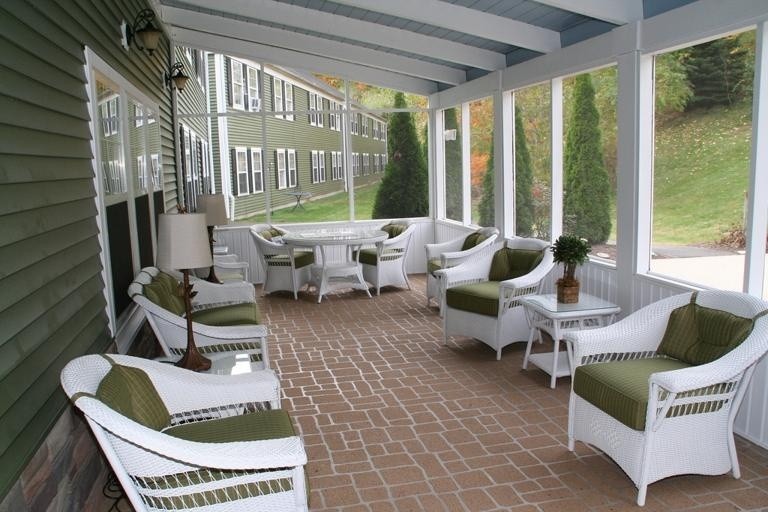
[550,233,592,304]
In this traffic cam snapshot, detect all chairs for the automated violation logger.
[433,236,561,361]
[60,352,311,511]
[196,254,250,282]
[424,226,500,318]
[127,266,268,361]
[352,218,416,296]
[563,288,767,507]
[249,223,318,301]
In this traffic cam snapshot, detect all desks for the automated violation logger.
[283,191,311,212]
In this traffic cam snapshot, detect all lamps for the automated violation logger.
[154,211,216,373]
[164,61,190,93]
[126,8,163,56]
[194,193,236,284]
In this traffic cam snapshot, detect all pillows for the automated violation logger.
[69,352,172,433]
[259,228,280,242]
[657,291,765,365]
[489,239,548,281]
[381,223,406,239]
[460,232,487,250]
[131,267,187,317]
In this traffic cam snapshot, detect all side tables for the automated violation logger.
[151,349,270,374]
[521,290,622,389]
